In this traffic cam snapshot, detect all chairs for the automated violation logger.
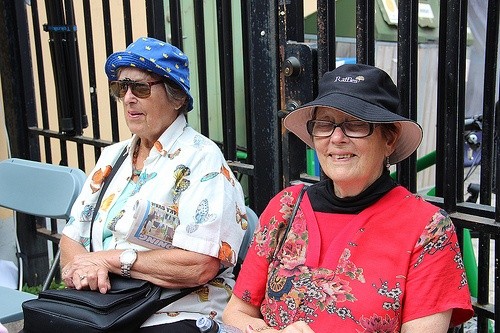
[0,157,87,333]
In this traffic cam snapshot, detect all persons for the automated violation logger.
[57,37,248,333]
[218,64,453,333]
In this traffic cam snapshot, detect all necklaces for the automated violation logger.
[132,139,142,176]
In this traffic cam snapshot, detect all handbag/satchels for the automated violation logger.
[22,272,162,333]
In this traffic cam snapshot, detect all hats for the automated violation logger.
[283,64,424,165]
[104,36,193,114]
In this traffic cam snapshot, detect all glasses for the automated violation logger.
[306,119,383,138]
[110,79,167,98]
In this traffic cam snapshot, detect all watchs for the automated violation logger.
[118,248,139,277]
